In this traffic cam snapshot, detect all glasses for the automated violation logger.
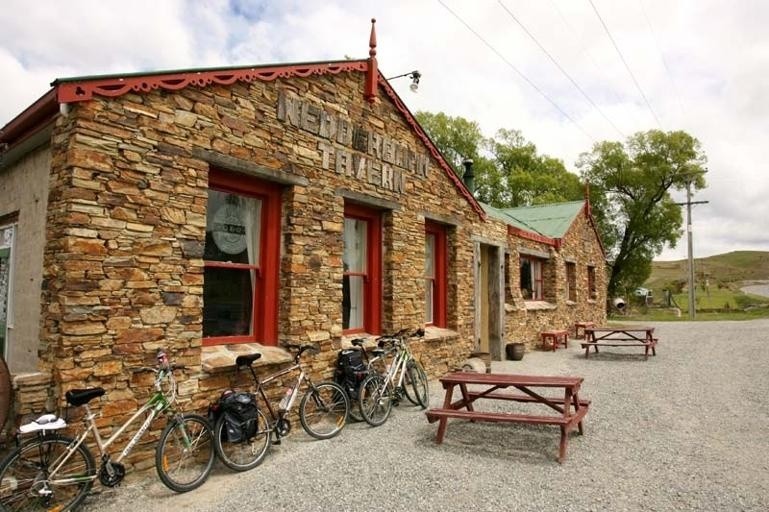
[30,406,60,425]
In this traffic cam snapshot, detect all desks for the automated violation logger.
[584,326,655,360]
[438,372,586,464]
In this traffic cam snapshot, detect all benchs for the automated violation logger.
[575,323,594,340]
[581,338,658,360]
[541,330,567,352]
[424,391,590,464]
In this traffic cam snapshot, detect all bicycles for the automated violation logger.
[215,342,351,472]
[358,328,430,427]
[1,364,216,512]
[342,327,426,422]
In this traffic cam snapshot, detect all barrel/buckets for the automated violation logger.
[506,343,525,360]
[461,357,487,374]
[613,298,626,310]
[471,351,491,374]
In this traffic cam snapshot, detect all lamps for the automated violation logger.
[377,70,420,92]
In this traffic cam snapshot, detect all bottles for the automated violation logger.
[278,385,293,410]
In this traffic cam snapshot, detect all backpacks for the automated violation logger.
[215,391,259,444]
[338,350,367,396]
